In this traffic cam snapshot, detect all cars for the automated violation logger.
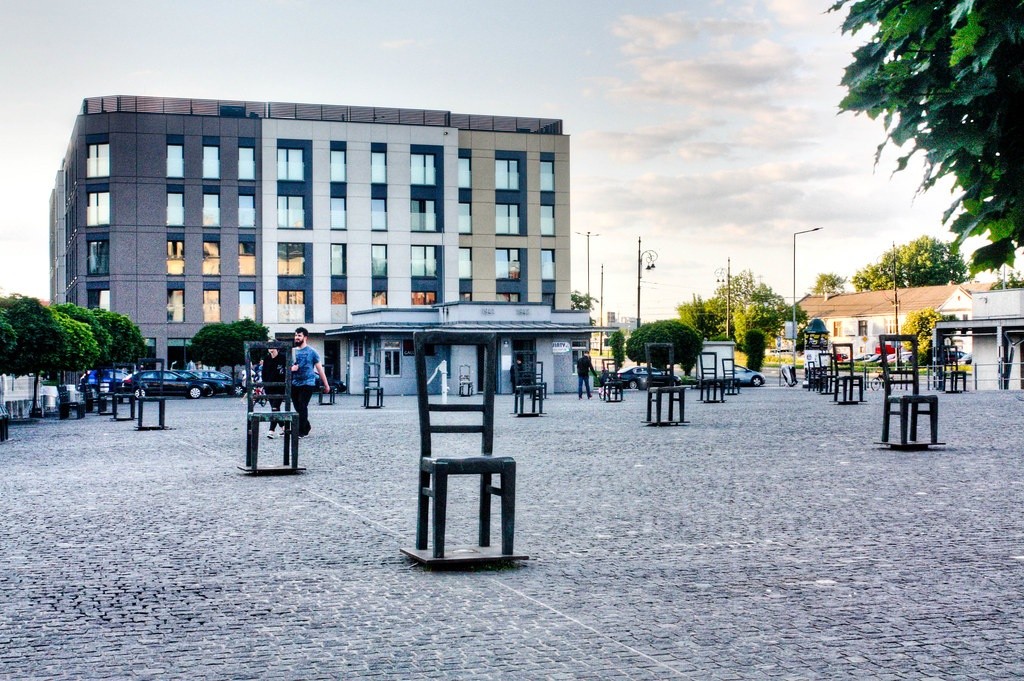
[614,366,682,389]
[845,348,974,375]
[122,369,246,399]
[77,370,130,392]
[313,372,347,394]
[734,365,766,387]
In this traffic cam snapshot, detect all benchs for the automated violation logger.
[58,383,107,420]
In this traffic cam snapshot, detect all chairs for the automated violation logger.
[243,341,299,470]
[513,350,547,415]
[97,358,166,430]
[722,358,740,395]
[700,352,724,401]
[319,364,336,404]
[644,343,686,423]
[807,335,967,445]
[364,362,383,408]
[602,359,623,401]
[412,330,516,558]
[459,364,473,396]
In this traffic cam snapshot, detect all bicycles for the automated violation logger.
[252,388,266,406]
[599,386,612,399]
[871,371,895,392]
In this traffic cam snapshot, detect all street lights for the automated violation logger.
[714,257,730,339]
[637,236,658,365]
[793,227,824,364]
[575,231,601,310]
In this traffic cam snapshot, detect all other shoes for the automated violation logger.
[267,432,274,439]
[280,433,285,436]
[299,427,312,439]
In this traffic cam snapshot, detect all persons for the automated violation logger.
[285,327,330,439]
[262,339,286,439]
[577,351,599,400]
[254,360,264,382]
[510,359,523,395]
[241,368,255,405]
